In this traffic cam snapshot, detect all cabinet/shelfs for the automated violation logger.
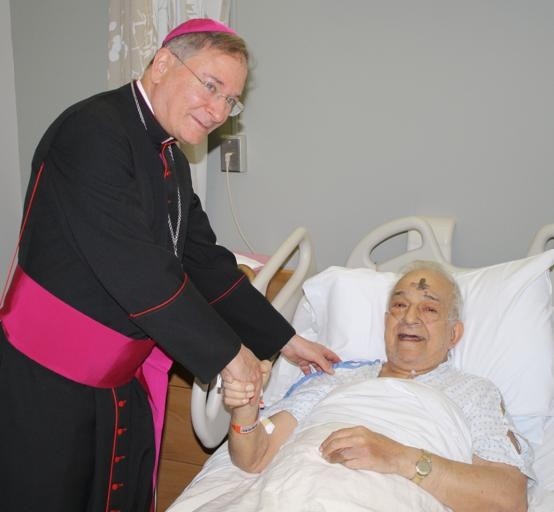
[149,266,294,512]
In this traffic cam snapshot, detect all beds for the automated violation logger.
[164,217,553,512]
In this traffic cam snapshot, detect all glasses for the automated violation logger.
[171,51,244,118]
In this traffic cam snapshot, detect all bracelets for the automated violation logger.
[228,420,261,434]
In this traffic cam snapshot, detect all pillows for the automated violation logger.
[302,244,553,440]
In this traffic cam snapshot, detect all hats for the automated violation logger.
[162,18,238,44]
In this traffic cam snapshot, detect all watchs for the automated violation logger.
[411,448,432,485]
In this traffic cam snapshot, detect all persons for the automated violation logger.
[220,260,537,511]
[0,18,340,511]
